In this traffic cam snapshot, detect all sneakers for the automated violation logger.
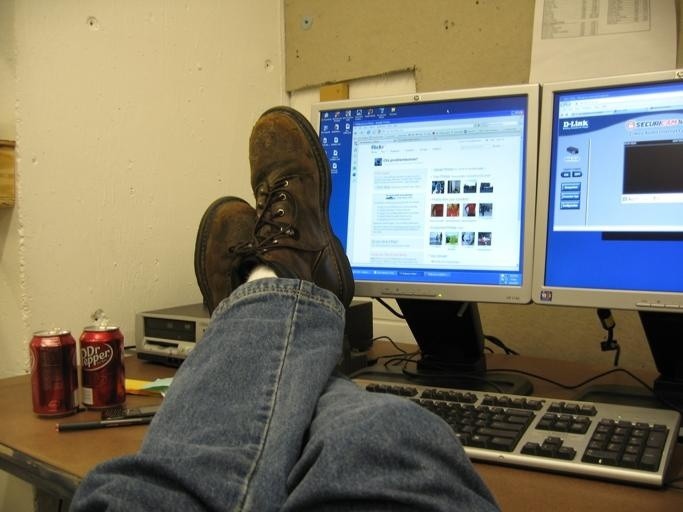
[229,107,354,310]
[194,197,260,316]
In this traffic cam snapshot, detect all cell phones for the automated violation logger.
[101,404,160,420]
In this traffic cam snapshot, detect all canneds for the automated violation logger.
[29,328,79,417]
[80,309,126,409]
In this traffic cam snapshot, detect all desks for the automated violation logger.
[0,336,680,512]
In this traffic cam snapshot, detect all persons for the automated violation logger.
[65,104,502,512]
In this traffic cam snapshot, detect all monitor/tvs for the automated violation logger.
[531,67,683,430]
[311,83,543,397]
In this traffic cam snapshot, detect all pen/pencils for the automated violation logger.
[56,416,153,432]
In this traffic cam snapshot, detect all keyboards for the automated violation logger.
[350,378,682,488]
